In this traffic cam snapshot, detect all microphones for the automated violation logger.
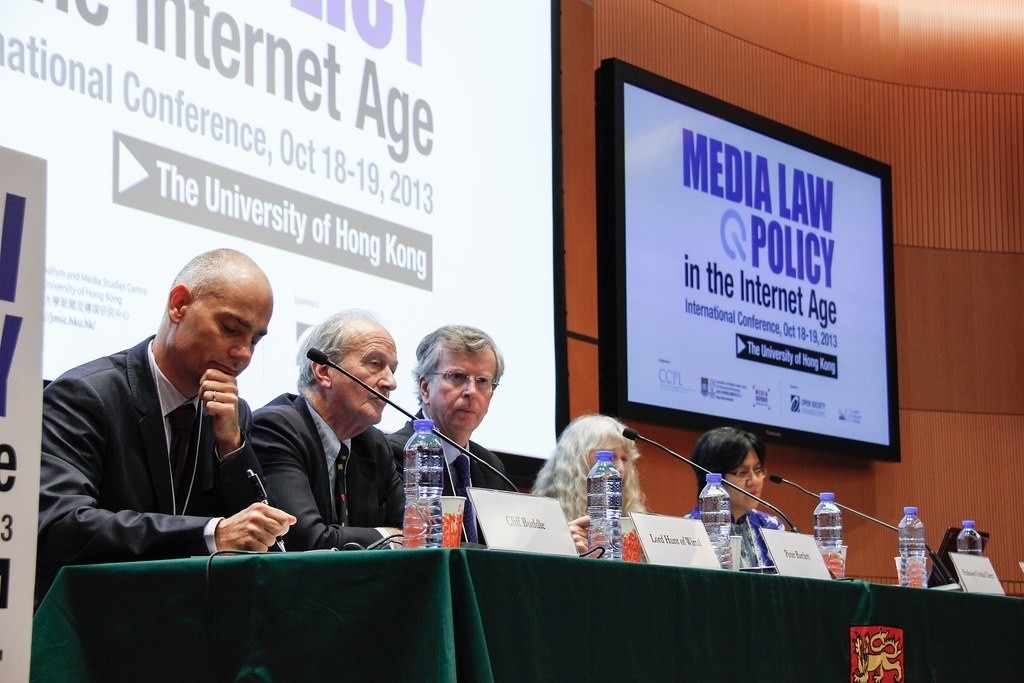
[623,428,800,532]
[767,474,963,590]
[305,347,520,492]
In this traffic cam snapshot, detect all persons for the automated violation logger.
[683,427,786,569]
[244,307,488,551]
[388,324,591,556]
[528,412,647,528]
[33,249,297,617]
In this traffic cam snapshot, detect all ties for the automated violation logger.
[169,405,196,495]
[335,445,350,529]
[454,455,479,544]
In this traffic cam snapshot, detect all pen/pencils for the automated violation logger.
[246,468,286,553]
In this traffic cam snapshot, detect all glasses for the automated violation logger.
[431,368,498,388]
[729,468,768,480]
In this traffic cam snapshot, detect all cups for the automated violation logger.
[894,557,928,585]
[841,546,848,567]
[729,536,743,572]
[440,496,467,548]
[620,517,643,562]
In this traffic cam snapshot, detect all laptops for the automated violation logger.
[927,528,989,588]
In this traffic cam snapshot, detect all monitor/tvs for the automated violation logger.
[594,57,902,464]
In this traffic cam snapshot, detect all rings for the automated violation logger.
[213,390,216,401]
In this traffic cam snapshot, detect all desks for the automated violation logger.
[31,548,1024,683]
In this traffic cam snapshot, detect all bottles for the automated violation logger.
[402,418,445,549]
[699,473,733,570]
[898,506,928,588]
[813,492,845,579]
[587,450,623,559]
[957,520,982,556]
